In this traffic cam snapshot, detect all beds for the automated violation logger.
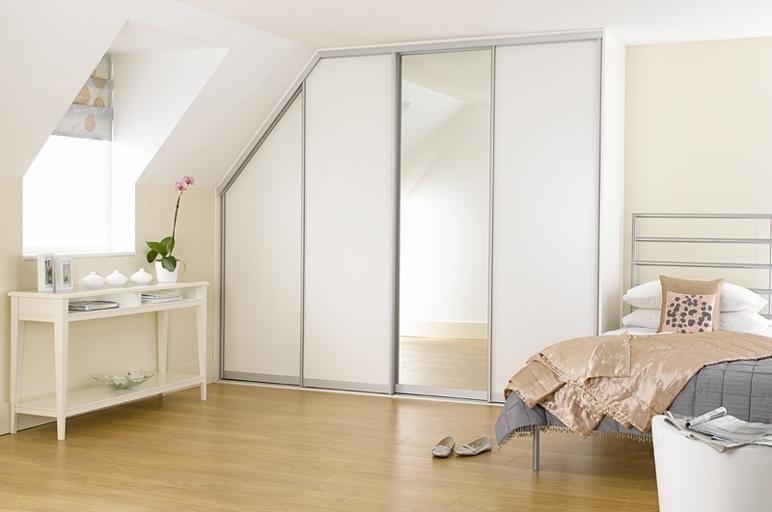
[496,212,772,471]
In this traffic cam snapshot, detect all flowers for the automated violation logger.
[146,175,195,271]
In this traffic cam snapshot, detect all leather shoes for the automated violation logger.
[454,436,491,456]
[431,435,456,458]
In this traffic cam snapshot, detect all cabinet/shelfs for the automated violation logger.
[8,280,210,441]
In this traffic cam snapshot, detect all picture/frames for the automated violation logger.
[59,257,74,290]
[37,253,55,291]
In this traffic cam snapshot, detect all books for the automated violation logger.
[663,406,771,453]
[69,301,124,311]
[143,292,186,304]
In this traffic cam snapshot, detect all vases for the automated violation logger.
[155,260,180,282]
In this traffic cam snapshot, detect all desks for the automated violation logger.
[651,415,772,512]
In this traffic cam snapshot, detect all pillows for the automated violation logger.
[621,276,772,334]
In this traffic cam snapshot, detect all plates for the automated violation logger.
[89,369,154,389]
